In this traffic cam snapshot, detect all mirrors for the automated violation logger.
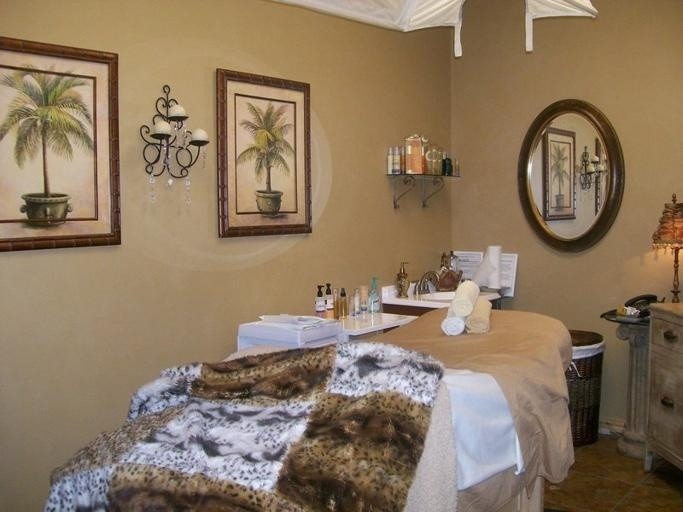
[515,98,626,253]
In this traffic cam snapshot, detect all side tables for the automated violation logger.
[338,312,419,341]
[600,307,665,462]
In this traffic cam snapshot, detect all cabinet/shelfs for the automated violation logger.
[644,301,683,472]
[238,316,350,351]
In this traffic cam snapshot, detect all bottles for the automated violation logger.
[439,250,457,271]
[333,276,380,320]
[387,134,461,177]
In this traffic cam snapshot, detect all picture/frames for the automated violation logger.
[594,136,608,216]
[215,67,313,238]
[0,36,122,252]
[542,126,577,220]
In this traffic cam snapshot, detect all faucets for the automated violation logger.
[420,271,440,290]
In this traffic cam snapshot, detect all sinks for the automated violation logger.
[418,291,456,300]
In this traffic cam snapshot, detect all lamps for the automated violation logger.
[579,146,604,191]
[140,84,210,209]
[650,192,683,302]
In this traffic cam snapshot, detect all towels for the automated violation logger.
[440,279,493,336]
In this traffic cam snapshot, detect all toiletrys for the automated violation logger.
[314,275,382,320]
[384,132,455,178]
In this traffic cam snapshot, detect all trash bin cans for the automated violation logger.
[565,329,606,446]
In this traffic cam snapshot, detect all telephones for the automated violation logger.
[625,295,666,318]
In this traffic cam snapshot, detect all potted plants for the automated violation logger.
[0,63,95,229]
[237,99,295,217]
[549,142,570,211]
